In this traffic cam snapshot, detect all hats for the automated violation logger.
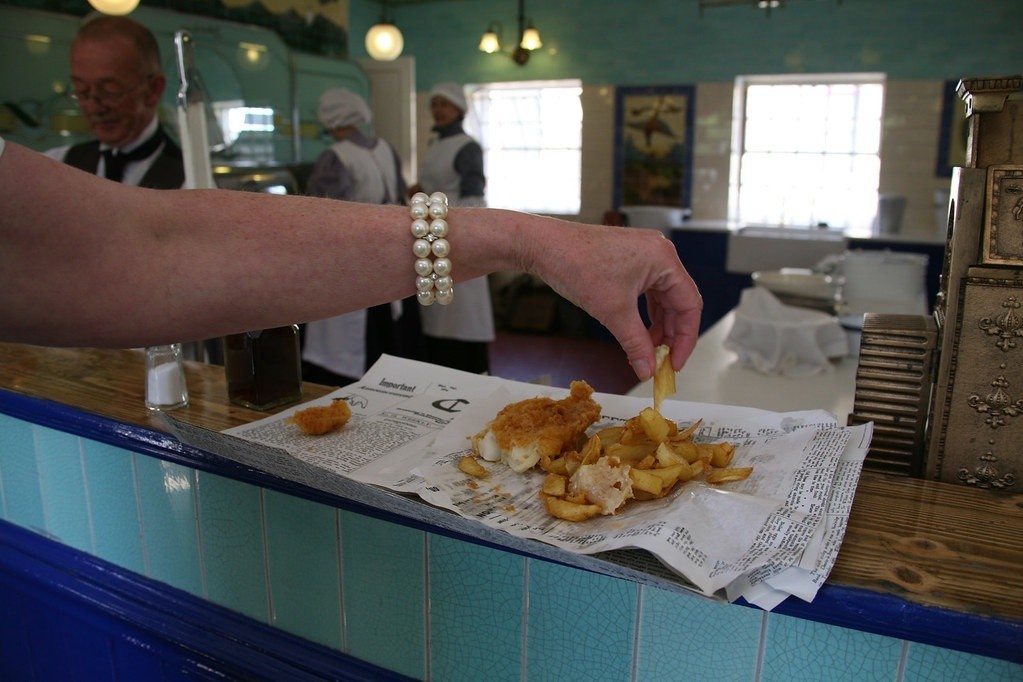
[316,88,371,130]
[428,80,468,113]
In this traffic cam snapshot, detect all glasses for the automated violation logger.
[63,74,152,106]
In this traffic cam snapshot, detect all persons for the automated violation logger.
[0,135,703,381]
[408,85,494,375]
[299,90,423,385]
[41,18,222,364]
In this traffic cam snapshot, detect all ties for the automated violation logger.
[100,122,165,182]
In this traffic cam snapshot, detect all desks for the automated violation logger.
[624,306,858,428]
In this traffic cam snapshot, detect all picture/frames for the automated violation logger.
[612,83,697,221]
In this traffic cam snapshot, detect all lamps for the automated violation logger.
[363,0,404,61]
[477,0,543,67]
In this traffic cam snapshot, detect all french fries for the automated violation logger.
[456,456,486,477]
[541,345,754,520]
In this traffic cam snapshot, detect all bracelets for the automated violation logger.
[410,191,454,306]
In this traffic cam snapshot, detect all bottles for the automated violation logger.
[224,324,303,411]
[143,343,189,411]
